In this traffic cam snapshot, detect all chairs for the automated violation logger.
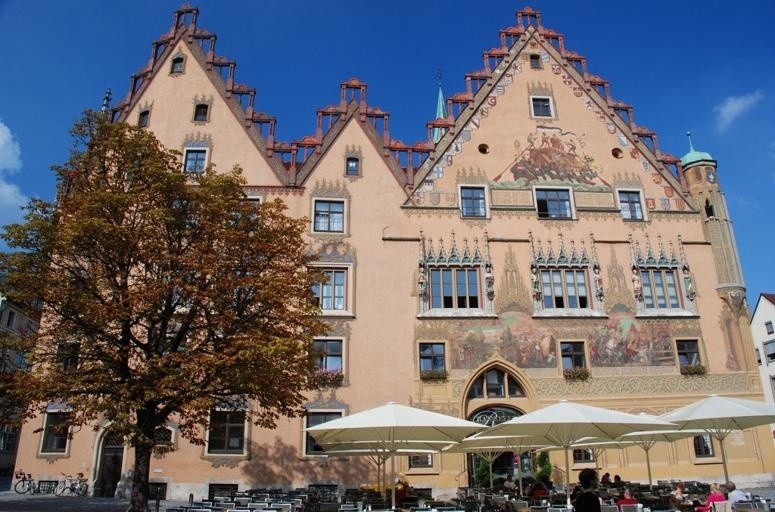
[171,490,774,512]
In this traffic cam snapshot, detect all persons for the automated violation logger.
[527,133,537,150]
[630,265,641,294]
[540,132,551,149]
[681,267,694,294]
[563,139,578,155]
[486,264,494,295]
[592,266,603,295]
[417,263,427,291]
[531,266,541,298]
[67,471,88,497]
[549,133,562,151]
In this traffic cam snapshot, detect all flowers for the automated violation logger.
[418,370,446,382]
[306,369,344,390]
[682,363,709,374]
[563,368,588,381]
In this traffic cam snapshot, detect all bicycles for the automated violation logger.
[54,472,88,496]
[14,469,36,494]
